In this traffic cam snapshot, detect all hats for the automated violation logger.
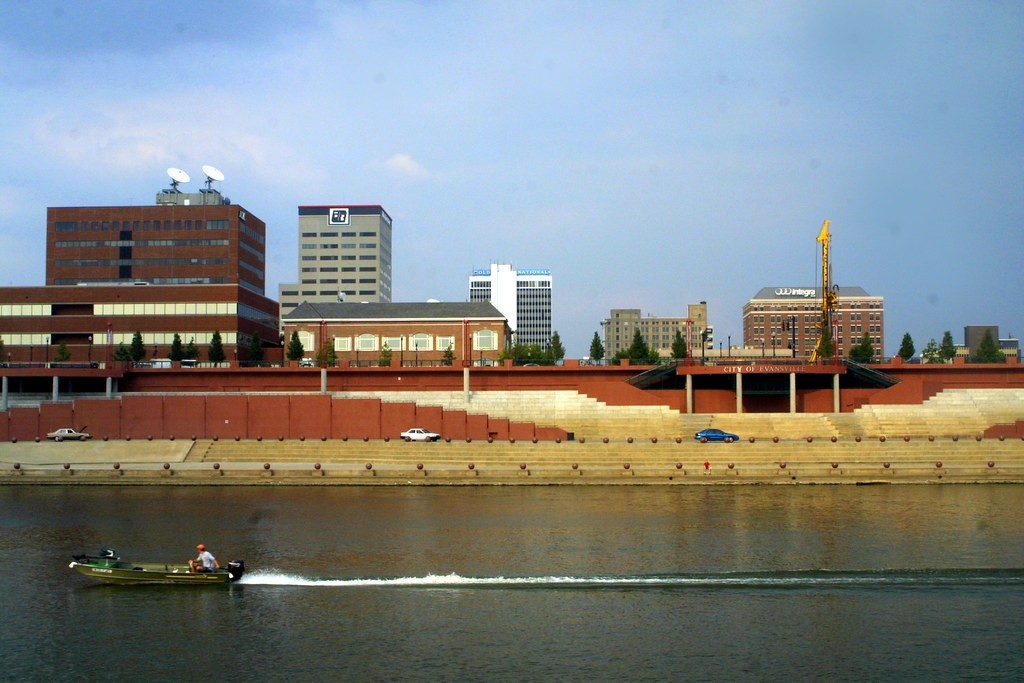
[196,545,205,549]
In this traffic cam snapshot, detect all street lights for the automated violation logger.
[720,339,722,358]
[700,331,704,358]
[762,338,765,358]
[728,332,731,358]
[772,331,776,358]
[88,336,92,361]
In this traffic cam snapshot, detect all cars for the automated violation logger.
[523,363,540,366]
[301,360,311,367]
[46,426,93,441]
[695,428,739,443]
[400,429,441,442]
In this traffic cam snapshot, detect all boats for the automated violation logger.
[68,547,246,587]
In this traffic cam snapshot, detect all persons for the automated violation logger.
[188,544,220,573]
